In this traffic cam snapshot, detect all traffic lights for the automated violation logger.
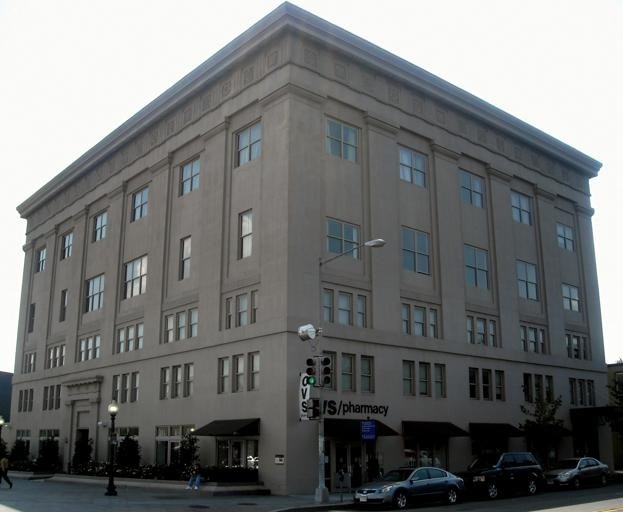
[305,358,319,386]
[318,355,331,387]
[307,399,320,420]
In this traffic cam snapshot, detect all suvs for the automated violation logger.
[462,450,545,499]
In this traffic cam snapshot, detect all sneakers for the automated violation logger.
[184,486,200,490]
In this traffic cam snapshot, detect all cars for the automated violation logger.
[546,456,610,489]
[354,466,465,507]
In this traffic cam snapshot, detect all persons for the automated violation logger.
[0,453,13,489]
[184,462,206,490]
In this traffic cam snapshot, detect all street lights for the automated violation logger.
[104,397,119,496]
[0,416,4,437]
[311,237,387,503]
